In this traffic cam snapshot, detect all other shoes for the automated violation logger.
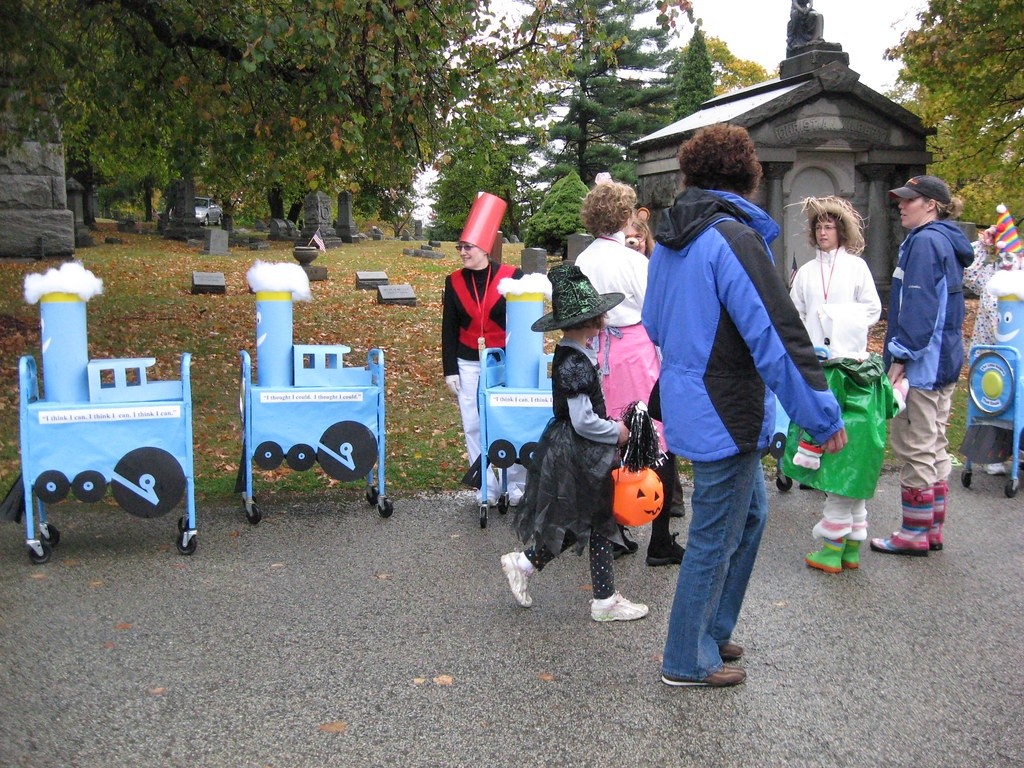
[477,488,500,506]
[646,532,686,566]
[669,503,685,517]
[984,462,1007,476]
[717,644,743,660]
[661,665,746,686]
[611,522,638,559]
[1017,448,1024,462]
[506,481,523,506]
[799,483,814,490]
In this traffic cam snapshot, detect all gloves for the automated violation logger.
[446,375,460,397]
[793,442,824,470]
[891,377,910,411]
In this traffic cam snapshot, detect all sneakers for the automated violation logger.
[501,552,533,608]
[590,589,649,623]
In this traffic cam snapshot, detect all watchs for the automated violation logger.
[889,356,905,365]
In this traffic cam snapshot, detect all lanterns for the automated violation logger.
[612,468,665,526]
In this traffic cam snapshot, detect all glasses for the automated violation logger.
[623,232,647,243]
[812,223,838,233]
[455,243,476,252]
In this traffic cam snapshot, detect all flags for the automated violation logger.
[312,229,326,252]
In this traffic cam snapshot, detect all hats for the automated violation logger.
[994,202,1024,256]
[888,175,951,205]
[531,264,625,332]
[459,191,508,257]
[804,193,866,257]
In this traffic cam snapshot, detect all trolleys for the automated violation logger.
[958,270,1024,497]
[760,344,830,493]
[233,259,395,526]
[0,261,198,563]
[460,269,557,530]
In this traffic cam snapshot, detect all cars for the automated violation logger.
[169,196,224,226]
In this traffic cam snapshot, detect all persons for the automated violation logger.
[441,241,524,508]
[639,123,849,687]
[871,175,975,555]
[960,224,1024,475]
[574,182,686,566]
[779,301,908,572]
[789,195,882,353]
[501,313,648,622]
[622,215,685,518]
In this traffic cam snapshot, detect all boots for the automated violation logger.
[841,539,862,569]
[805,537,845,573]
[870,482,934,557]
[929,480,948,551]
